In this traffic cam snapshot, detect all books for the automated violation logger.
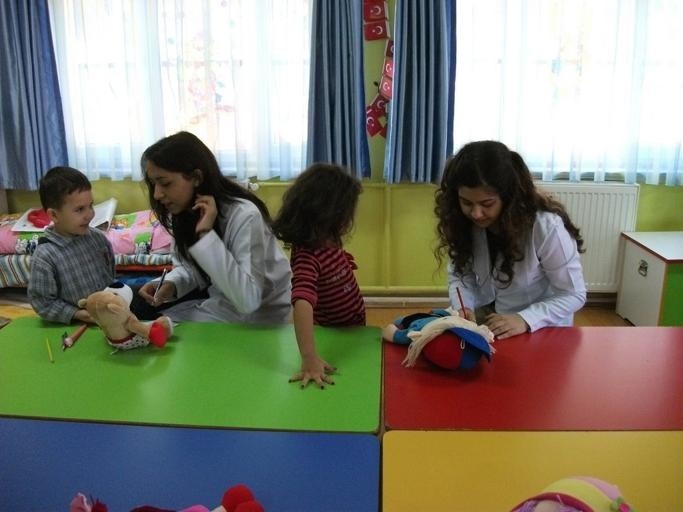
[10,197,117,233]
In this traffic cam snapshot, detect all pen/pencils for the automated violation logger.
[456,287,467,319]
[151,268,167,305]
[65,324,86,346]
[46,338,52,362]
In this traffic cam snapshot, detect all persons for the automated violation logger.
[27,166,117,326]
[432,140,588,341]
[137,131,293,327]
[266,161,368,391]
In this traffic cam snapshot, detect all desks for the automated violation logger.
[614,230,683,326]
[0,308,683,512]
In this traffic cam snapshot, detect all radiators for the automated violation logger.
[532,182,641,295]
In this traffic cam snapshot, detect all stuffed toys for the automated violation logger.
[509,477,634,512]
[78,280,174,351]
[69,483,265,512]
[382,306,500,373]
